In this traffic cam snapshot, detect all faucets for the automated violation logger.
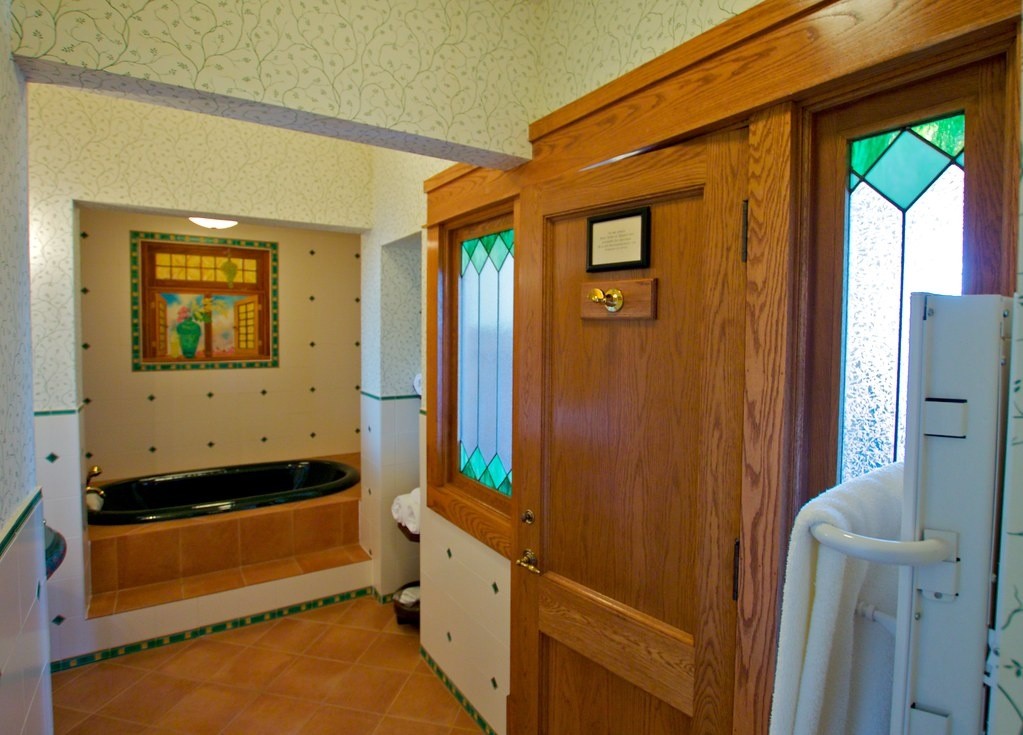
[85,465,108,501]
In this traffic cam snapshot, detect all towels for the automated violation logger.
[85,493,106,512]
[412,373,423,396]
[765,458,906,735]
[398,586,420,607]
[390,487,422,541]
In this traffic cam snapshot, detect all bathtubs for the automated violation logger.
[79,449,364,600]
